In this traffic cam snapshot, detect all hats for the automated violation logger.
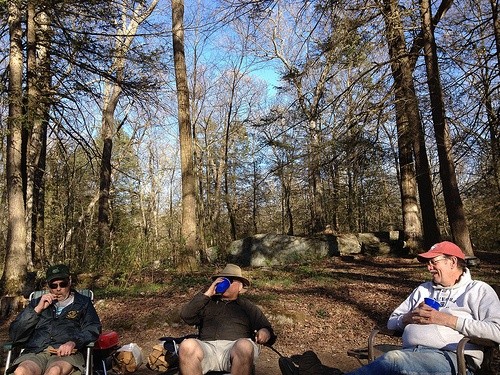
[417,241,465,267]
[46,264,70,283]
[212,264,251,289]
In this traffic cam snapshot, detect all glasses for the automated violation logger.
[49,281,69,289]
[230,279,240,284]
[423,257,446,266]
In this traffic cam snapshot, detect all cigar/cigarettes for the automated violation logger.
[54,294,59,297]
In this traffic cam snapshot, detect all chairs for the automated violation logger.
[2,289,94,375]
[158,325,257,375]
[369,285,500,375]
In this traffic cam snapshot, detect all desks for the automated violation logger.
[347,344,401,367]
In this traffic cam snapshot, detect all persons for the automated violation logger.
[339,241,499,375]
[178,264,278,375]
[9,264,102,375]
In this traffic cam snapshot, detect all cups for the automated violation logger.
[424,298,442,311]
[216,278,231,293]
